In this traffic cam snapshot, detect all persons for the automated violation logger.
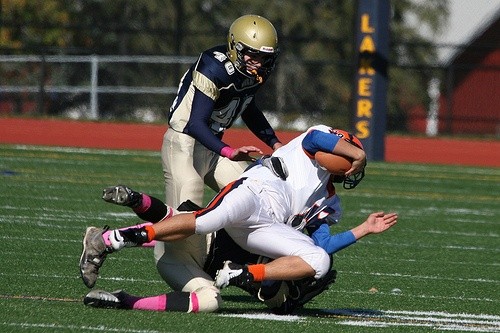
[79,14,398,312]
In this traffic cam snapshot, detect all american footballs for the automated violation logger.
[315,150,352,175]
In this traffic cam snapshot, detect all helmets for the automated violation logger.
[228,15,277,78]
[329,129,363,184]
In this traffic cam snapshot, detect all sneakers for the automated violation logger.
[102,186,140,208]
[212,260,246,290]
[79,226,107,287]
[84,289,124,308]
